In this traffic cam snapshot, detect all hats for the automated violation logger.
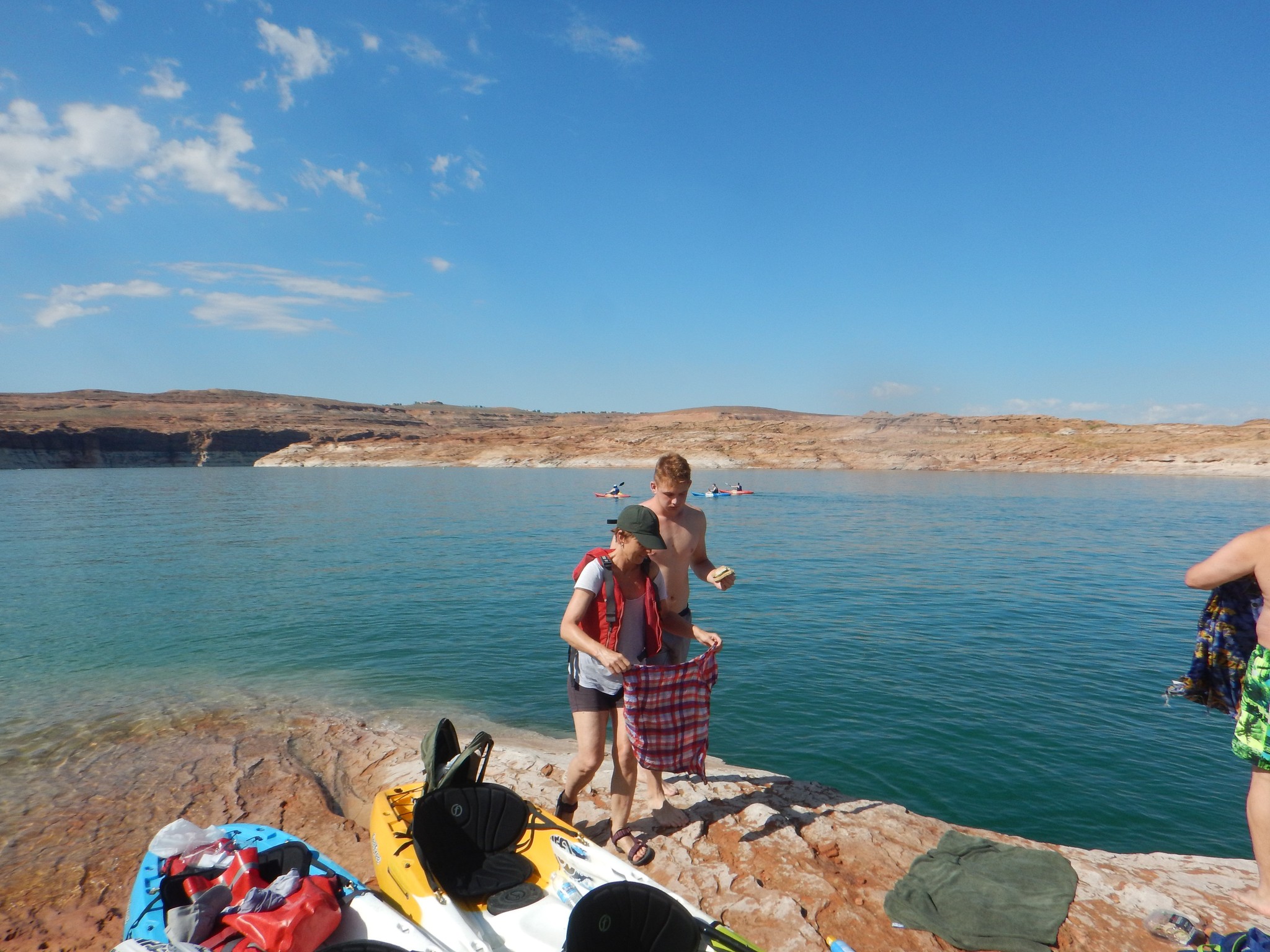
[607,504,667,550]
[736,483,740,485]
[613,484,618,487]
[163,886,233,946]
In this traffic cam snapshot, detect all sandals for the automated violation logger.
[553,789,578,829]
[610,826,652,866]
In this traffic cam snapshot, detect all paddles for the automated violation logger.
[550,834,756,952]
[708,488,712,492]
[602,482,625,497]
[107,938,213,952]
[725,483,737,490]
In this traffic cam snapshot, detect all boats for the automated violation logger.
[121,822,451,952]
[691,491,731,496]
[369,778,764,952]
[718,489,754,494]
[593,492,631,498]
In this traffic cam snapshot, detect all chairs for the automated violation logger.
[160,838,312,910]
[412,783,535,904]
[562,880,702,952]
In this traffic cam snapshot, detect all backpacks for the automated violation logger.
[420,716,494,791]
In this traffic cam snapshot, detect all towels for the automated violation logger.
[620,642,718,786]
[881,830,1079,952]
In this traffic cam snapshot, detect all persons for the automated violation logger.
[1184,523,1270,916]
[554,504,722,867]
[609,451,736,828]
[706,484,718,494]
[606,485,619,495]
[731,483,742,491]
